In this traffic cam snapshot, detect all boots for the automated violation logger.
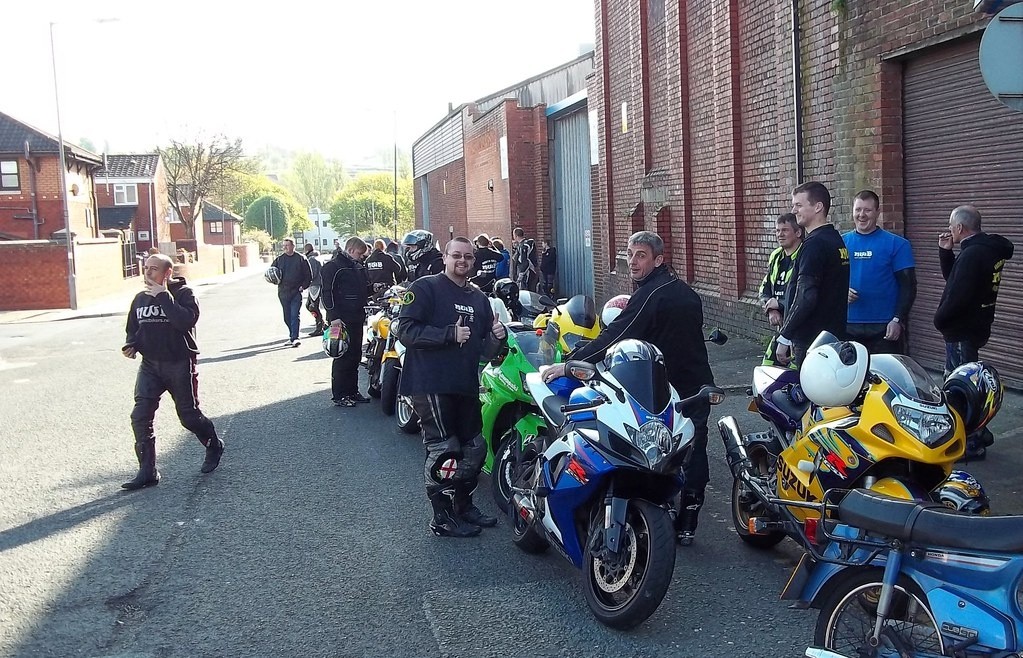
[197,421,225,473]
[453,477,497,527]
[308,318,327,336]
[659,497,677,521]
[673,488,703,546]
[426,482,482,537]
[123,437,160,489]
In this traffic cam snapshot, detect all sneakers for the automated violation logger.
[349,390,370,403]
[333,396,356,407]
[284,340,301,347]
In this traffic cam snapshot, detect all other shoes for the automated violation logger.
[951,432,986,462]
[977,428,993,447]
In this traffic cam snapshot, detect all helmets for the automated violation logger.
[403,229,433,261]
[264,266,282,285]
[933,468,990,515]
[493,278,519,308]
[600,294,632,329]
[943,361,1003,434]
[322,320,350,359]
[800,330,870,408]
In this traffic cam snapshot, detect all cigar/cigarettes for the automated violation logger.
[945,236,953,240]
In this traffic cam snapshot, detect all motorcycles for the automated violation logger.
[357,282,602,516]
[504,338,727,631]
[715,353,967,619]
[779,488,1023,658]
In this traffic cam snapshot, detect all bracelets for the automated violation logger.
[893,317,905,331]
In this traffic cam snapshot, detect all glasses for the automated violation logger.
[446,253,474,260]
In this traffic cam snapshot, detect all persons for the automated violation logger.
[933,205,1014,463]
[512,228,538,290]
[776,182,850,368]
[271,239,312,347]
[305,243,328,337]
[541,230,716,546]
[540,240,556,299]
[469,235,510,298]
[759,213,803,366]
[403,230,446,282]
[397,236,509,538]
[363,239,407,294]
[121,254,225,489]
[333,241,342,257]
[319,237,382,407]
[840,190,917,355]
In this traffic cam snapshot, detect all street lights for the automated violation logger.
[48,17,122,310]
[269,199,279,262]
[220,154,259,275]
